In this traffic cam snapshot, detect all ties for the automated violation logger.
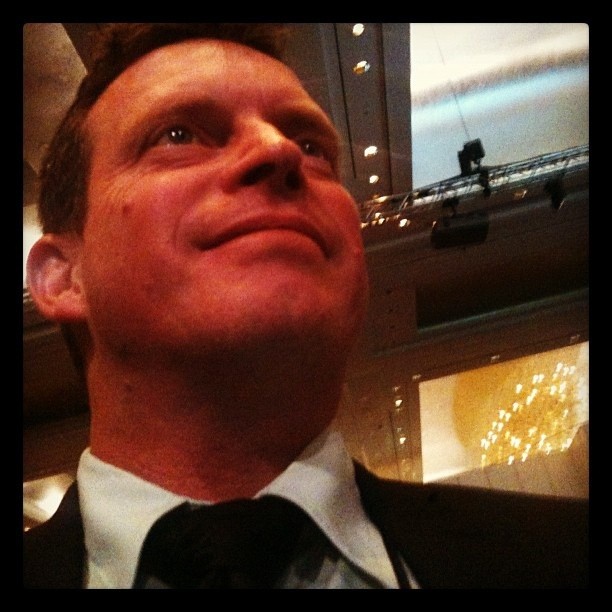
[141,498,319,595]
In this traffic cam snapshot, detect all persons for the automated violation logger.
[24,25,594,592]
